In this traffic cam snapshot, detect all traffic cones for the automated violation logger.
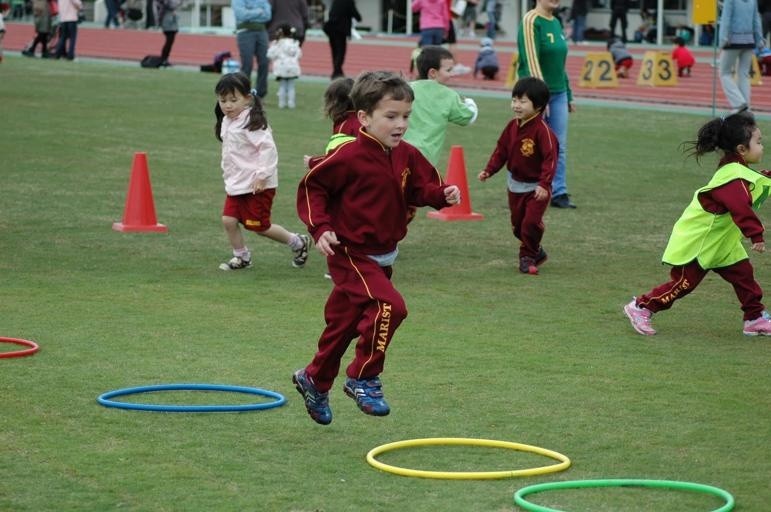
[107,149,168,234]
[426,141,485,223]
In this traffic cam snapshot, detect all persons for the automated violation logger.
[398,46,479,169]
[476,77,561,275]
[0,0,84,59]
[291,68,461,428]
[213,71,312,271]
[303,77,363,169]
[230,0,500,110]
[102,0,126,28]
[717,0,764,114]
[621,104,771,337]
[157,0,179,66]
[567,0,696,77]
[516,0,577,210]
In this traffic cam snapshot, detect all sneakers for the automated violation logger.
[623,298,657,335]
[343,370,390,416]
[518,249,548,275]
[292,234,312,269]
[220,253,254,271]
[551,192,577,208]
[292,367,334,426]
[743,311,771,336]
